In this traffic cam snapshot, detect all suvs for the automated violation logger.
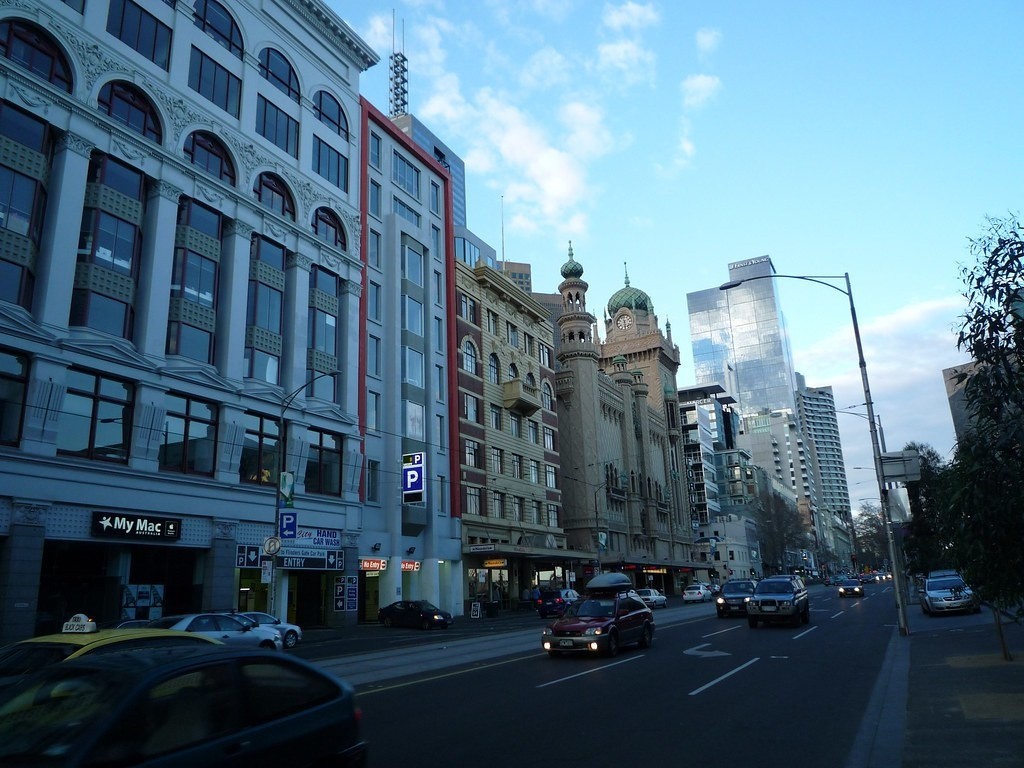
[713,578,760,619]
[538,589,580,619]
[746,576,810,628]
[541,573,657,658]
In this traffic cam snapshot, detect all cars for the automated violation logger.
[378,600,454,630]
[238,612,303,647]
[0,641,371,768]
[682,584,713,602]
[826,572,892,586]
[917,568,981,616]
[104,614,283,651]
[707,584,720,596]
[0,614,286,742]
[636,589,668,610]
[838,579,865,597]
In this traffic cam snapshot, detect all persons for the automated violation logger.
[522,586,553,608]
[494,586,508,610]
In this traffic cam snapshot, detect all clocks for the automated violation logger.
[616,314,632,331]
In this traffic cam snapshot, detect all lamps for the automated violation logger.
[406,547,416,556]
[372,542,381,552]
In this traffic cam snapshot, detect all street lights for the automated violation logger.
[266,369,342,616]
[718,271,910,635]
[594,472,637,574]
